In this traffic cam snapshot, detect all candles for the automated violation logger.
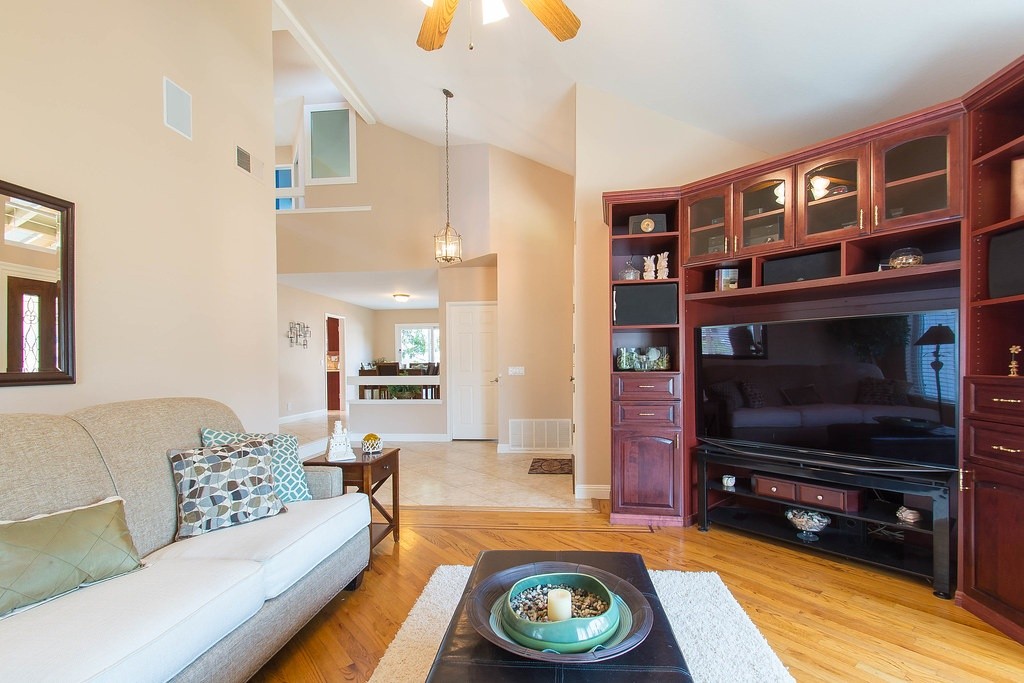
[548,589,572,620]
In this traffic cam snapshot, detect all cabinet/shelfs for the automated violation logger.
[601,54,1024,644]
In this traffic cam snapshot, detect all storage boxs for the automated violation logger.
[614,284,678,325]
[629,214,667,236]
[715,269,738,291]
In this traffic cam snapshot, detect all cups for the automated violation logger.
[722,474,735,487]
[616,347,672,371]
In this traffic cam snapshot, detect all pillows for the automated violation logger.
[720,374,914,407]
[0,425,313,622]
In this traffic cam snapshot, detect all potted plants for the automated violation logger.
[385,372,421,399]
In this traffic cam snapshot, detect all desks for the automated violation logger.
[826,422,957,465]
[358,367,425,399]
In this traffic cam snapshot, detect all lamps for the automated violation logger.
[287,321,312,348]
[912,324,955,425]
[774,172,829,205]
[393,294,410,302]
[434,91,463,264]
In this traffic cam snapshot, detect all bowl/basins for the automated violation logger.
[888,247,925,269]
[502,573,620,652]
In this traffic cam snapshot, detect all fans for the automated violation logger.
[417,0,583,52]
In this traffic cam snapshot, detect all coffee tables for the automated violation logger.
[425,548,694,683]
[302,448,400,571]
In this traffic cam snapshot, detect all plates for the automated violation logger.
[466,562,654,663]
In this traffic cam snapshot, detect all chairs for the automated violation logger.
[375,362,440,399]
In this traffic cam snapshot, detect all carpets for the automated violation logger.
[366,564,798,683]
[528,457,572,475]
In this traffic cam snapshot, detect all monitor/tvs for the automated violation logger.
[695,307,959,471]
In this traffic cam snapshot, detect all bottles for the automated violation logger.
[618,261,641,280]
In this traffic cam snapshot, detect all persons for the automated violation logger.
[729,326,761,356]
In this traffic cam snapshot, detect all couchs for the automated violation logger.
[700,361,942,445]
[0,398,371,683]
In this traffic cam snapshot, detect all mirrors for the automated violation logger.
[0,179,76,384]
